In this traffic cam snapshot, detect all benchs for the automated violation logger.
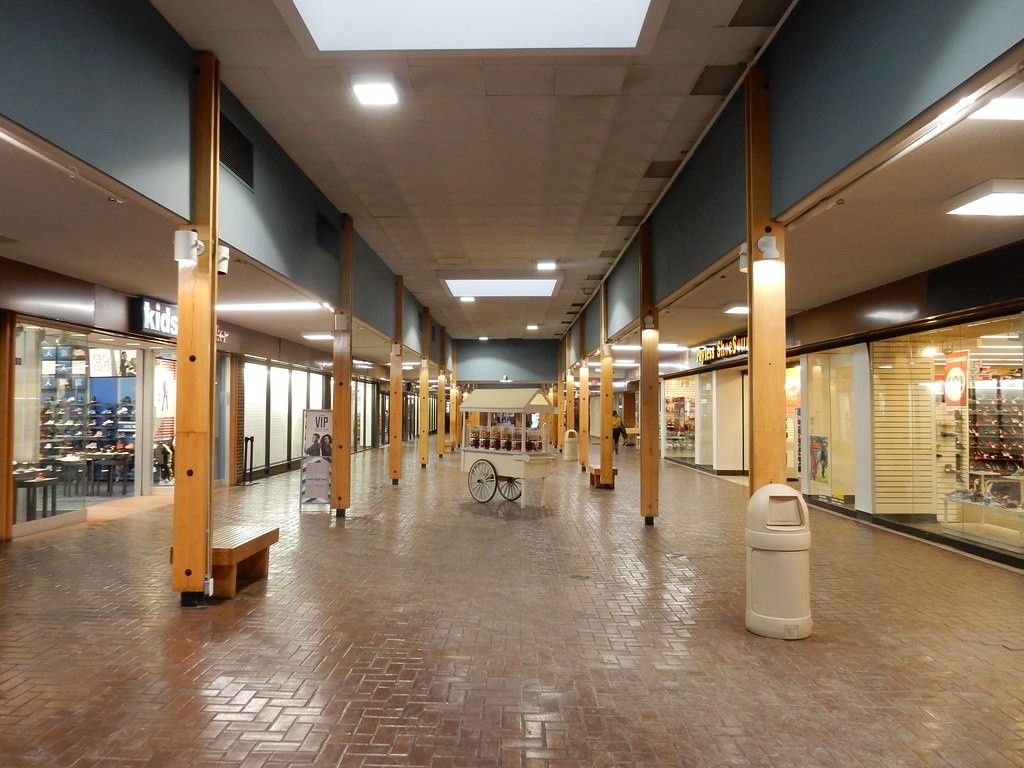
[168,524,280,598]
[587,463,618,489]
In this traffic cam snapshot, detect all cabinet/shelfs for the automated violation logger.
[940,383,1024,481]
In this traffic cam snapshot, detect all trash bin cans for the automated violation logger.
[742,481,815,641]
[563,429,579,461]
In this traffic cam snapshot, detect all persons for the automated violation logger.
[612,410,626,455]
[161,380,169,411]
[320,434,332,456]
[119,351,134,377]
[809,436,829,481]
[154,439,175,483]
[303,433,321,456]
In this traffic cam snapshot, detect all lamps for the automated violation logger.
[216,244,230,276]
[421,359,427,367]
[603,342,611,356]
[738,242,748,273]
[334,313,347,329]
[645,314,656,329]
[391,343,401,357]
[758,235,780,260]
[172,230,205,266]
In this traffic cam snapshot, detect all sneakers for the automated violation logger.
[936,387,1023,516]
[11,390,135,486]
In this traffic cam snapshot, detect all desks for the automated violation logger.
[73,449,130,495]
[38,454,86,495]
[14,475,59,523]
